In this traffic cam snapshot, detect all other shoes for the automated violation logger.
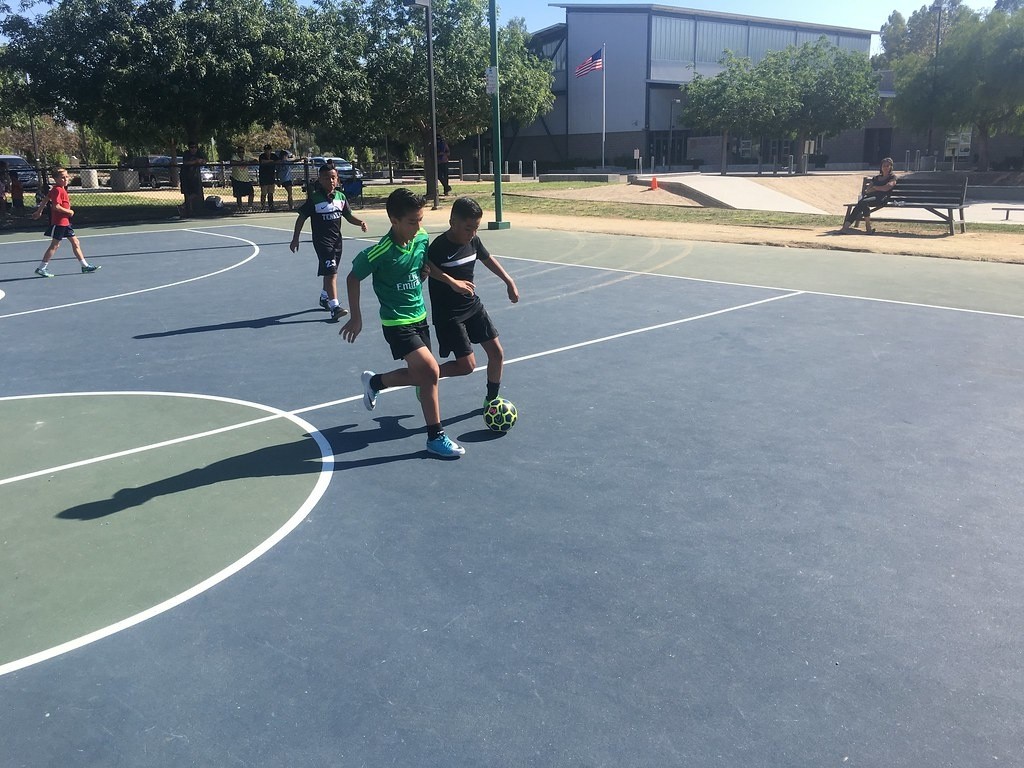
[483,396,505,409]
[416,386,421,401]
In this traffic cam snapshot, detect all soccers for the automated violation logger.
[484,398,518,433]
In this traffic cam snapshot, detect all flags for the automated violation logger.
[574,47,603,78]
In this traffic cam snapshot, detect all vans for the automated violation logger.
[0,155,38,191]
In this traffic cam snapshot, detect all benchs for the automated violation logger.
[842,175,971,236]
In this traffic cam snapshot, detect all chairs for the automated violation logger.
[343,179,363,209]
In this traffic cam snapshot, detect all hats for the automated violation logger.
[281,150,289,155]
[263,145,273,150]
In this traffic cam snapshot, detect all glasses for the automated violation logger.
[238,151,244,154]
[189,146,197,149]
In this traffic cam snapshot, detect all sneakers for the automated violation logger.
[427,435,466,458]
[79,264,102,273]
[36,268,52,277]
[330,307,349,321]
[361,371,381,410]
[320,296,330,309]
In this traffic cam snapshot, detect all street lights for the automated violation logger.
[403,0,442,211]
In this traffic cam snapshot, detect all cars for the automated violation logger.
[147,157,212,189]
[118,155,160,182]
[215,150,363,187]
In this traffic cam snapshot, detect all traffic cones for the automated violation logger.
[649,177,661,191]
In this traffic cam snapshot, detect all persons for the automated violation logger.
[176,142,207,217]
[834,157,898,235]
[0,161,26,228]
[258,144,282,211]
[276,151,305,210]
[230,146,259,210]
[416,196,519,409]
[30,168,101,278]
[430,134,452,196]
[290,164,366,320]
[338,188,466,457]
